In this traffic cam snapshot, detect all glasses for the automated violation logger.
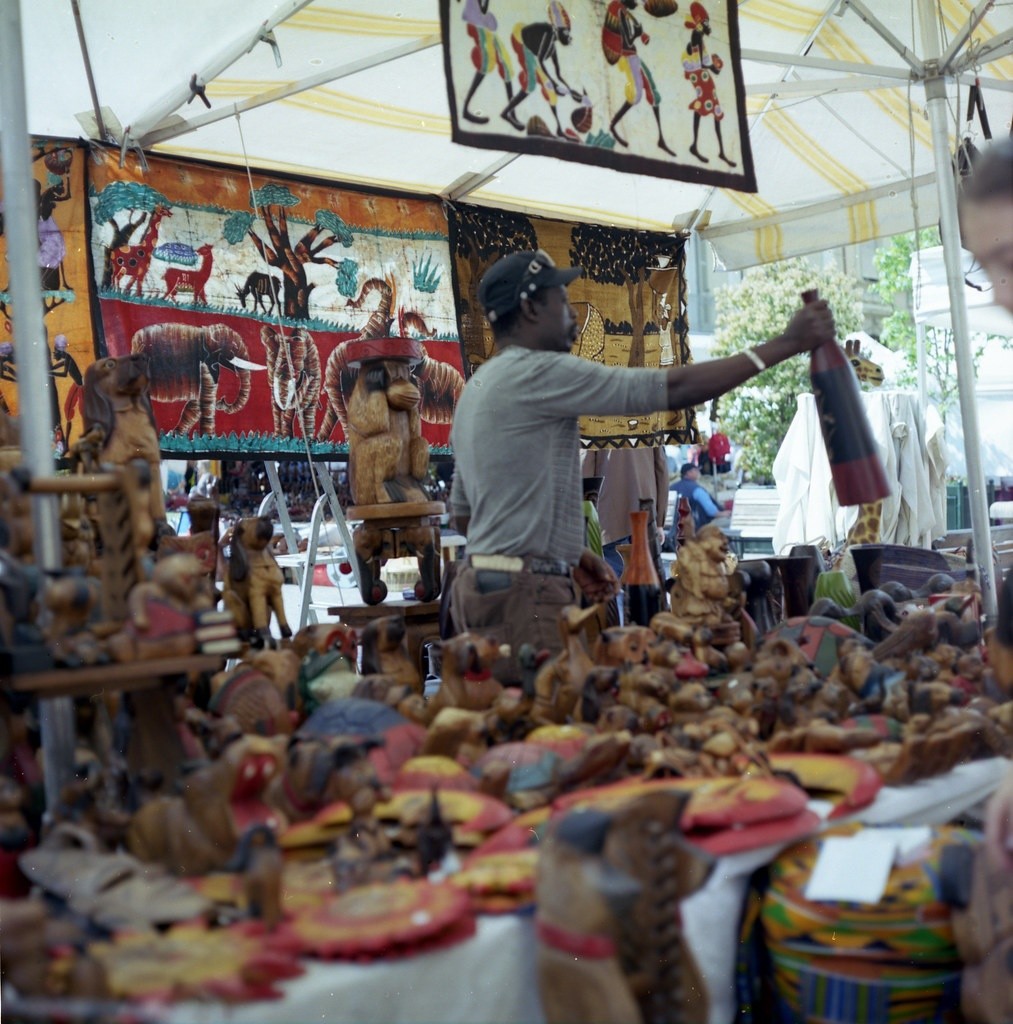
[513,246,556,305]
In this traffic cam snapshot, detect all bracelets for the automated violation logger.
[742,348,764,373]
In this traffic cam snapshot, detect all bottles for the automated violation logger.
[620,511,661,625]
[639,498,667,613]
[802,289,891,505]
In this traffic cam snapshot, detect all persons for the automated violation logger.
[579,447,669,579]
[446,249,837,642]
[955,130,1013,872]
[669,463,732,535]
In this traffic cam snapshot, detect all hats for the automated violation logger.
[475,243,579,324]
[681,463,702,477]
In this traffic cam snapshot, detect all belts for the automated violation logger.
[468,554,571,577]
